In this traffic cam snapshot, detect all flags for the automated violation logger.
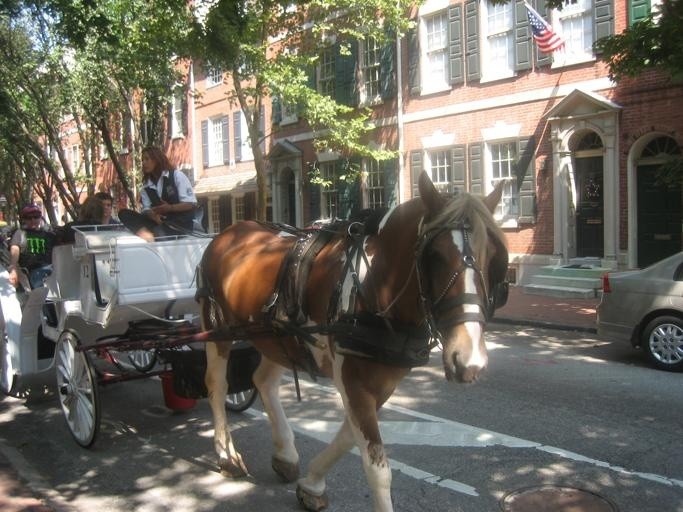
[528,9,566,57]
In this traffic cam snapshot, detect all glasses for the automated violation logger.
[22,215,41,220]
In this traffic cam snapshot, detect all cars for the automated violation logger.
[593,250,683,369]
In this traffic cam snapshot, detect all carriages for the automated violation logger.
[0,171,510,512]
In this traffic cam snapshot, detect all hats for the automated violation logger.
[19,206,43,215]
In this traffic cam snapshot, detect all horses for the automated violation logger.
[195,169,507,512]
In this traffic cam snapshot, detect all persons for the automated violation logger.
[96,192,124,231]
[29,196,112,326]
[117,145,198,243]
[8,206,55,288]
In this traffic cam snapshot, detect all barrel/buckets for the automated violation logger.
[159,372,198,411]
